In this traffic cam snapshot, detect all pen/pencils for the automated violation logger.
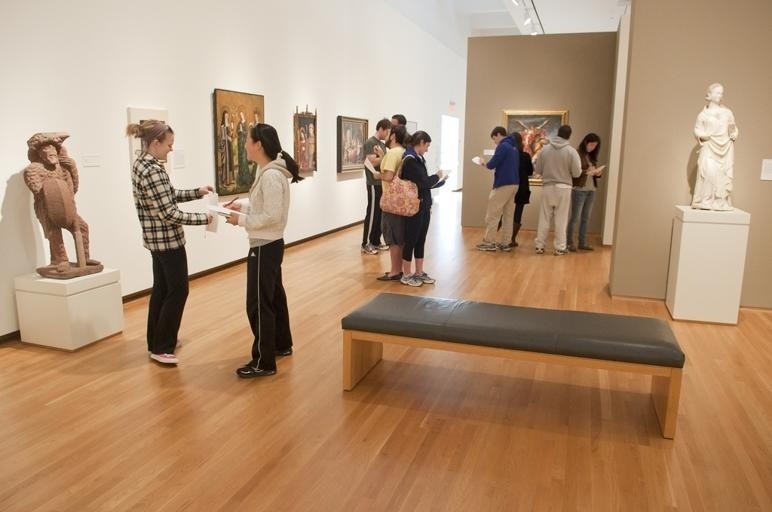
[223,197,240,208]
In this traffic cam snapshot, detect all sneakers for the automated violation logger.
[567,244,576,251]
[414,272,435,284]
[151,353,178,364]
[273,347,292,356]
[237,367,276,379]
[379,176,421,217]
[476,242,496,251]
[579,245,593,250]
[401,273,423,287]
[553,249,568,256]
[370,242,389,250]
[535,248,544,254]
[361,245,378,254]
[376,272,403,281]
[496,243,511,251]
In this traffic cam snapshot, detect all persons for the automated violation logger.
[132,120,215,364]
[237,111,253,185]
[298,123,315,169]
[361,120,391,254]
[400,131,448,286]
[692,81,739,210]
[254,111,260,129]
[377,124,406,281]
[223,124,293,378]
[535,125,582,254]
[24,144,98,272]
[567,132,603,251]
[391,115,411,147]
[497,132,533,247]
[476,127,520,251]
[220,110,234,185]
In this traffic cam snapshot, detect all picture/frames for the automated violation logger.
[211,87,271,197]
[290,104,320,178]
[334,114,370,174]
[502,108,570,188]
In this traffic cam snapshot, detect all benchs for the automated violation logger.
[330,289,685,443]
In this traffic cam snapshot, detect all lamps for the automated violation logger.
[512,0,539,36]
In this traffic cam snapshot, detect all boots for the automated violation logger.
[510,223,521,247]
